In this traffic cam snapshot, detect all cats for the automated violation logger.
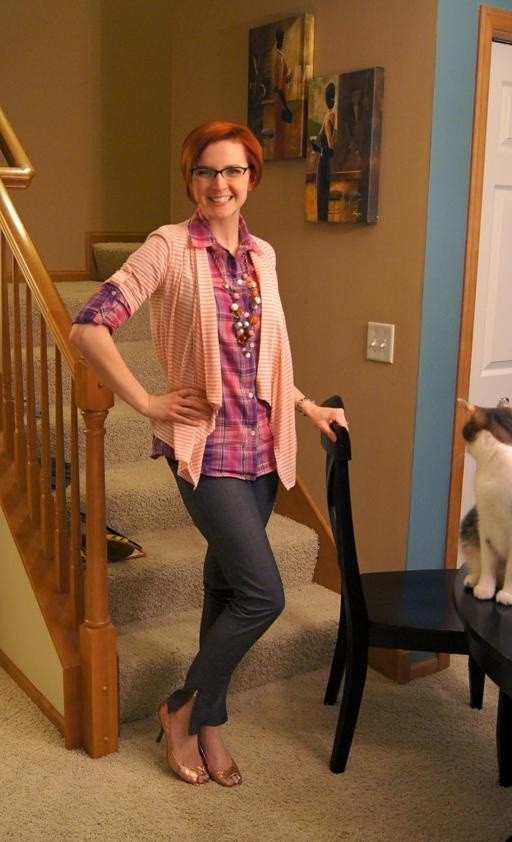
[461,397,512,606]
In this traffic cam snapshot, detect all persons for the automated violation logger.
[68,116,353,787]
[271,23,293,162]
[309,79,339,224]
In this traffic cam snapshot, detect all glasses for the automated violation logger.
[189,165,253,181]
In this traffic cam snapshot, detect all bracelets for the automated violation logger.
[295,397,308,416]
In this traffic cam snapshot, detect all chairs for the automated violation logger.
[320,395,487,774]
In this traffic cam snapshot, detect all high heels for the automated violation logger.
[155,693,242,786]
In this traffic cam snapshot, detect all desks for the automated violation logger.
[452,562,512,789]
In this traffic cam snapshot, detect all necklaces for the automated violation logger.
[208,249,261,358]
